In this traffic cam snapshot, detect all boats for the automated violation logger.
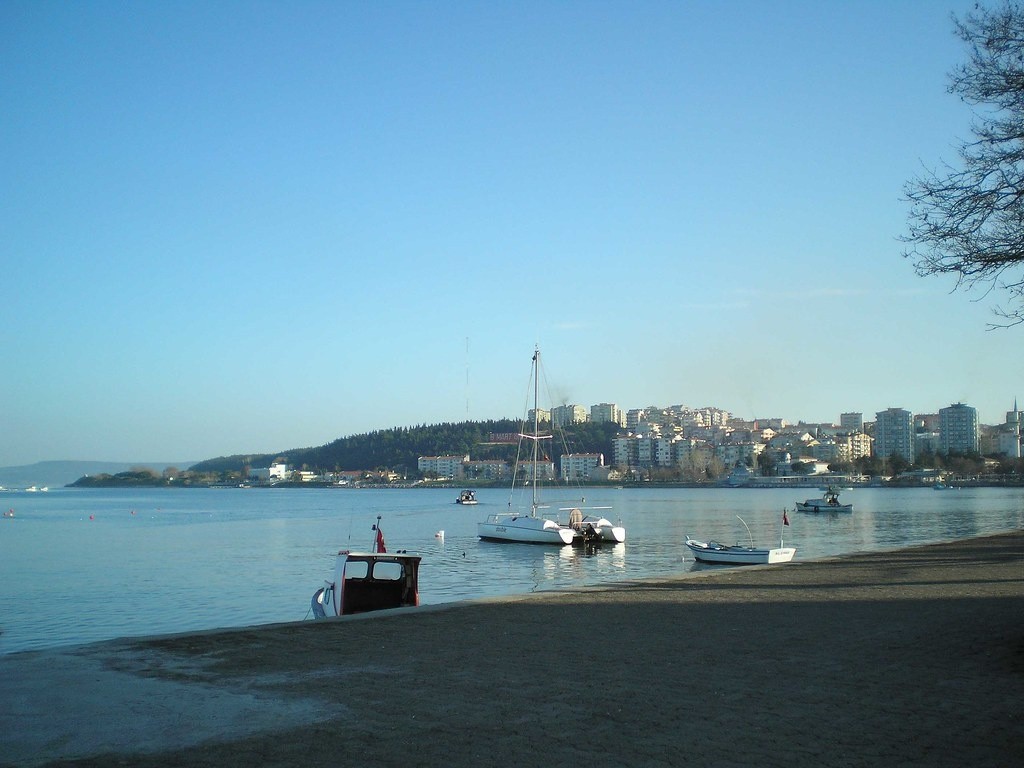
[795,484,853,512]
[311,516,422,621]
[684,507,796,564]
[455,488,478,504]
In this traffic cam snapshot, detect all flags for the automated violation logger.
[374,526,386,553]
[783,508,789,526]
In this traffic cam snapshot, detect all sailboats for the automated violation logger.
[476,342,626,545]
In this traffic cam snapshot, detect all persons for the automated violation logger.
[828,500,831,503]
